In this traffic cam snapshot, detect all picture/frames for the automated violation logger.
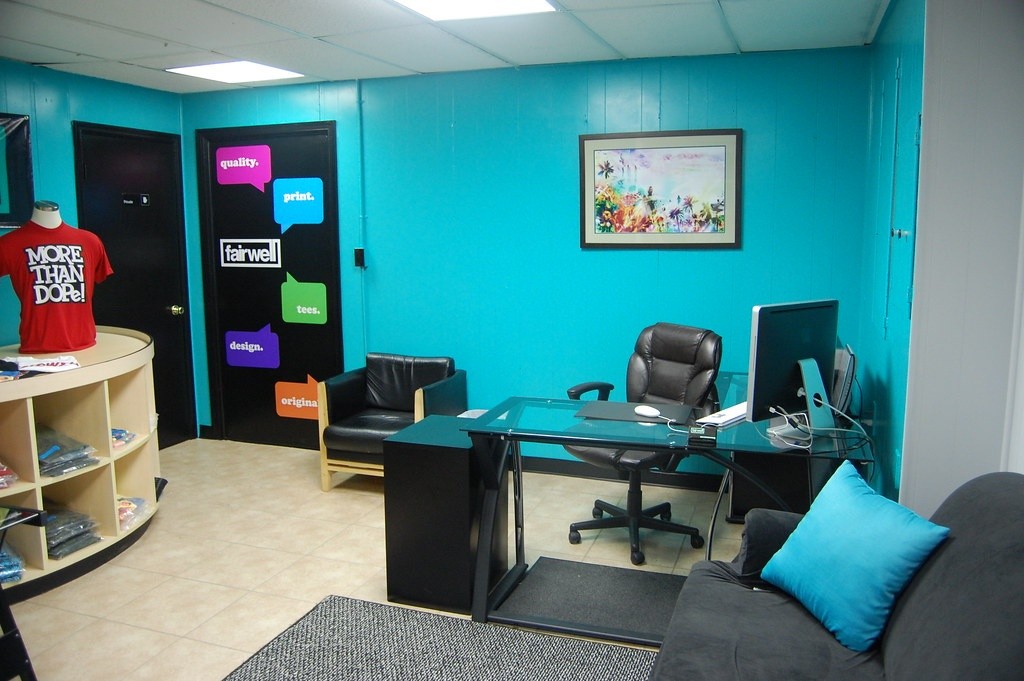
[579,128,743,249]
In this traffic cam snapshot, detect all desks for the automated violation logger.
[458,396,874,647]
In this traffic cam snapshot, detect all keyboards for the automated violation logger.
[695,402,747,426]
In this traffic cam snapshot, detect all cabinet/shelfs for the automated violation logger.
[382,414,511,614]
[726,380,811,524]
[0,326,159,607]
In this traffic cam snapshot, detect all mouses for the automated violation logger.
[634,405,660,417]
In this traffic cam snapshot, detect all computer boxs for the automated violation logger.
[727,450,834,524]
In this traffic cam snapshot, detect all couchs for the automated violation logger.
[647,470,1024,681]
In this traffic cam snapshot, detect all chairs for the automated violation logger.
[318,352,467,492]
[562,322,723,565]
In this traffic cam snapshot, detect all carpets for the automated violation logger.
[221,595,658,681]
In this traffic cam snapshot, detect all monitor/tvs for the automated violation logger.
[747,297,839,440]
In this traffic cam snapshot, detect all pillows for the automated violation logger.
[759,460,950,653]
